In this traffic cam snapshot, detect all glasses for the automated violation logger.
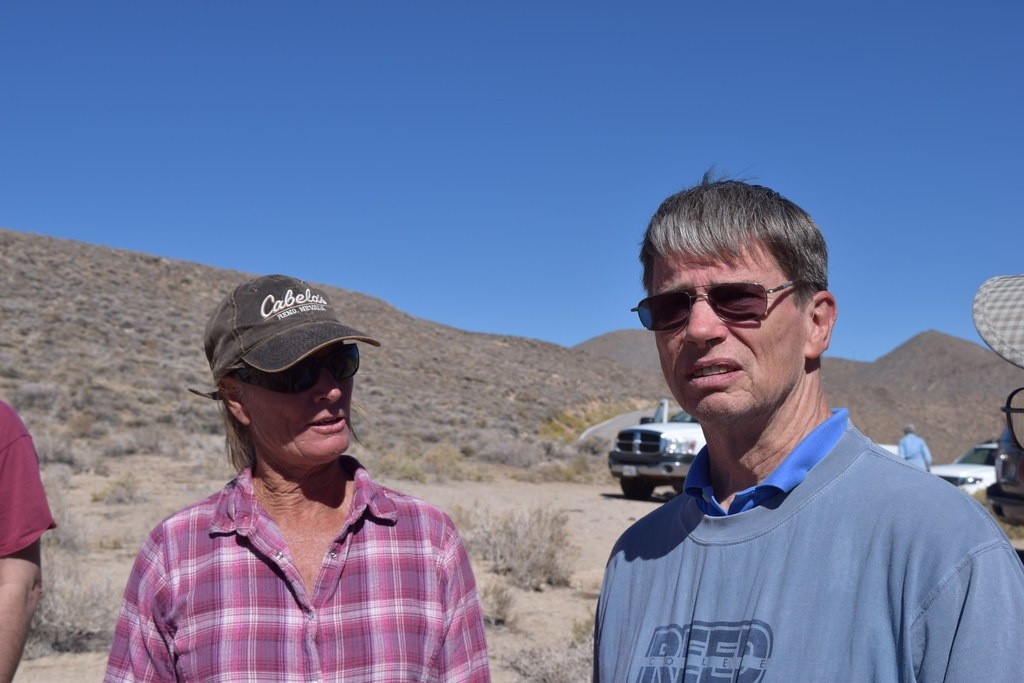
[226,343,360,394]
[631,278,802,332]
[1000,387,1024,450]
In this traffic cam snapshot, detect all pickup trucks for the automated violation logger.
[609,392,898,503]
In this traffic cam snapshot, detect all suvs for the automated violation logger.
[930,437,1002,496]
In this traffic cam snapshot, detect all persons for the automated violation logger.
[95,277,491,683]
[592,175,1024,683]
[1,400,57,683]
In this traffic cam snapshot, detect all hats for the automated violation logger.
[973,274,1024,369]
[187,274,381,400]
